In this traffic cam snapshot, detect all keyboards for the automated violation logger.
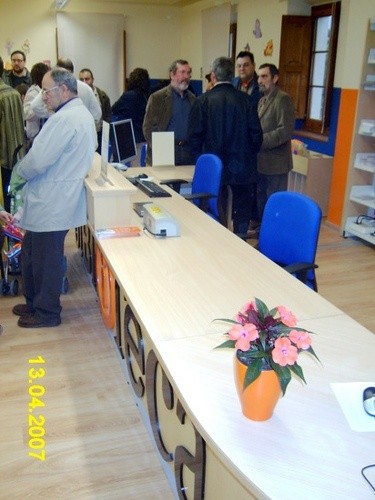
[136,181,171,197]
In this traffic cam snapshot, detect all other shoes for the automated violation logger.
[245,227,258,238]
[249,220,259,230]
[18,313,61,328]
[12,302,30,315]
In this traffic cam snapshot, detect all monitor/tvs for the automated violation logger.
[101,121,110,179]
[109,119,137,163]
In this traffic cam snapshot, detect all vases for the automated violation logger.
[234,350,286,422]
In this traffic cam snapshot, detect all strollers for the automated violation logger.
[0,143,69,298]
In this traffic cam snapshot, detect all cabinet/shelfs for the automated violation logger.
[337,17,375,245]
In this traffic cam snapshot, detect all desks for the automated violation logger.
[292,150,334,217]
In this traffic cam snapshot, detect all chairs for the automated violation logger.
[161,153,223,219]
[254,191,322,291]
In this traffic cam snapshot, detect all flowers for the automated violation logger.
[209,298,321,396]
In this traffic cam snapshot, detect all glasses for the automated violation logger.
[41,83,69,95]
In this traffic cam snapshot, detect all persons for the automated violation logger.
[256,63,295,248]
[231,50,263,105]
[109,68,151,143]
[142,59,197,192]
[188,55,263,241]
[205,74,212,92]
[13,68,98,328]
[0,51,111,217]
